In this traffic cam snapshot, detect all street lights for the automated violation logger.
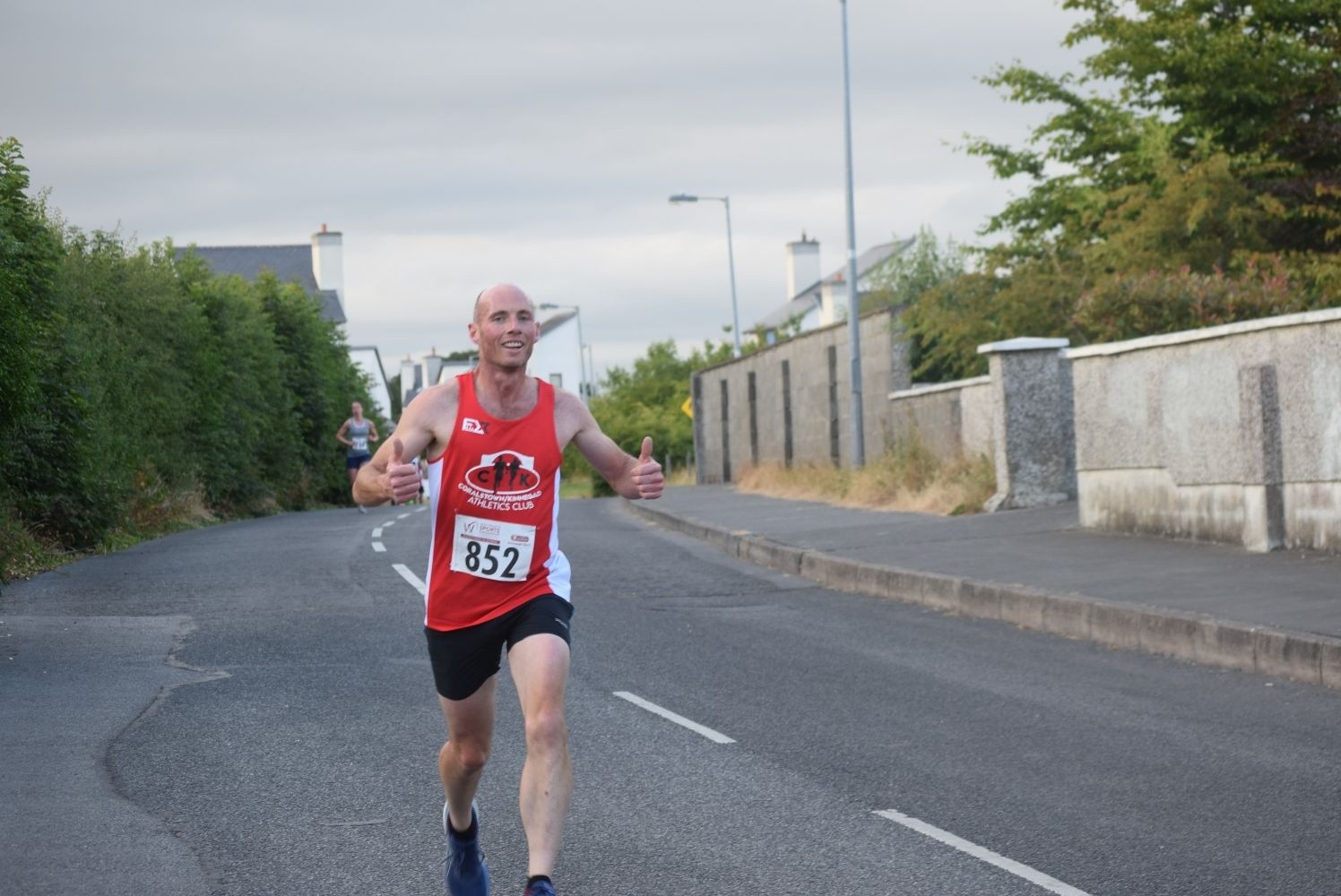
[669,194,742,360]
[536,301,591,416]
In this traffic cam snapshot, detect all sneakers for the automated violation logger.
[523,880,556,896]
[443,800,489,896]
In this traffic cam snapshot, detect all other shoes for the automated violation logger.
[358,507,366,513]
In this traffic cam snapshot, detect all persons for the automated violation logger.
[337,402,378,514]
[353,284,664,896]
[387,432,426,505]
